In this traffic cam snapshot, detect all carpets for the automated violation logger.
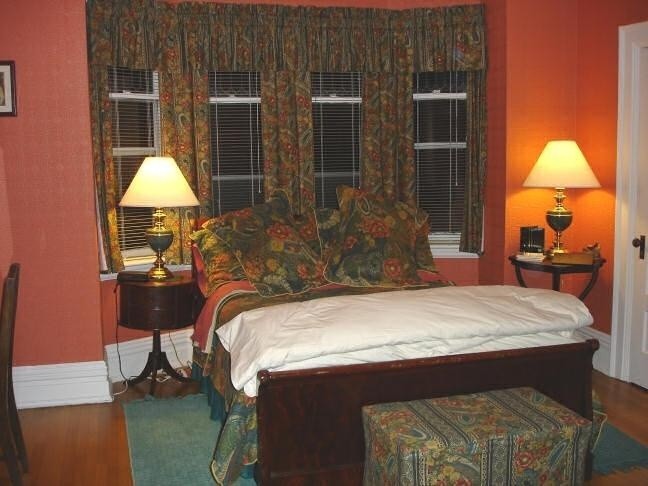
[123,399,647,486]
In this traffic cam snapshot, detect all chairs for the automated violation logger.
[0,262,30,486]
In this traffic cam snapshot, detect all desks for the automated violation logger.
[116,280,205,397]
[507,252,607,301]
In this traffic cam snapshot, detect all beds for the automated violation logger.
[190,205,599,485]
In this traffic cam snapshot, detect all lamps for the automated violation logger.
[118,156,202,280]
[521,139,602,258]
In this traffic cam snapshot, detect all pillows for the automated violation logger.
[190,185,439,297]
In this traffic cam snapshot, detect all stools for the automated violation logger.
[362,386,594,485]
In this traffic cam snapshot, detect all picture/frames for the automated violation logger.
[0,58,17,118]
